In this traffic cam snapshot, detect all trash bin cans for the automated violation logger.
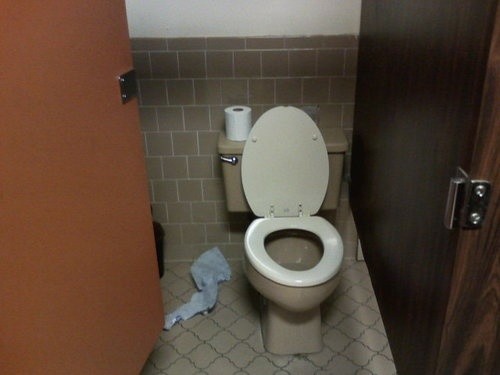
[152,221,166,278]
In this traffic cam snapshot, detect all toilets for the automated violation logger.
[216,105,350,355]
